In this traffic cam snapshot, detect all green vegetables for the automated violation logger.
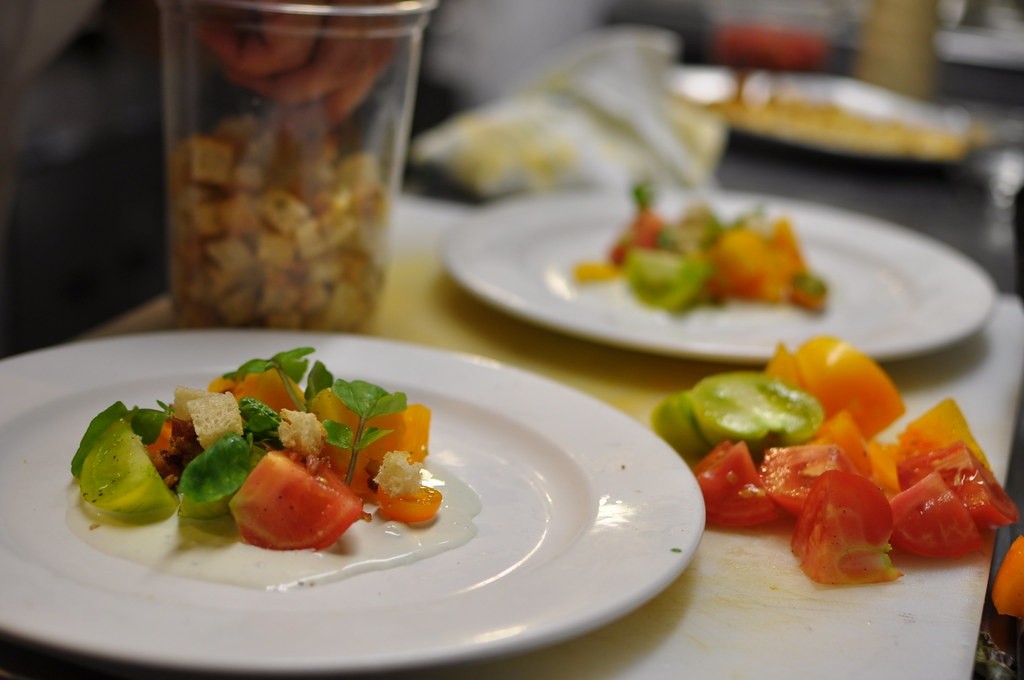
[69,344,407,506]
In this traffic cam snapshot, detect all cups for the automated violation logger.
[854,1,935,98]
[160,2,441,333]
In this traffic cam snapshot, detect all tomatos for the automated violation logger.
[650,334,1024,621]
[79,366,443,553]
[607,207,830,316]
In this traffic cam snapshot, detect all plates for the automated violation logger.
[935,26,1024,66]
[444,186,995,365]
[1,333,704,674]
